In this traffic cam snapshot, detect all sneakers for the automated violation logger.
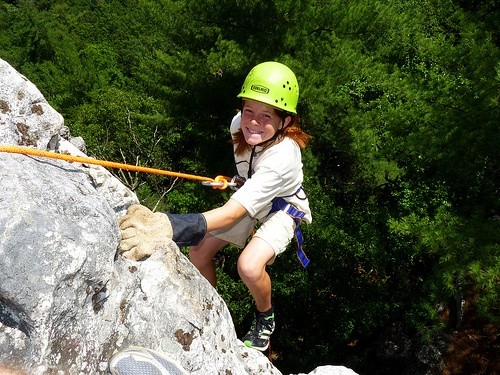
[108,347,187,375]
[242,305,275,350]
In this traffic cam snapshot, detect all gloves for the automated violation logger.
[119,205,207,260]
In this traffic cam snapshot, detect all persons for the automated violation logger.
[117,62,313,353]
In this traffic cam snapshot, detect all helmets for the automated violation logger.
[237,60,299,114]
